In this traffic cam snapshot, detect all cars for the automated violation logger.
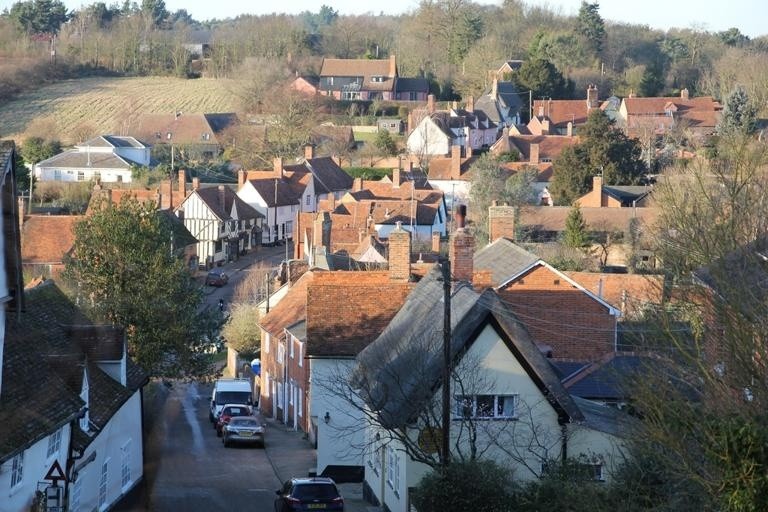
[275,474,346,511]
[208,375,267,449]
[205,270,229,288]
